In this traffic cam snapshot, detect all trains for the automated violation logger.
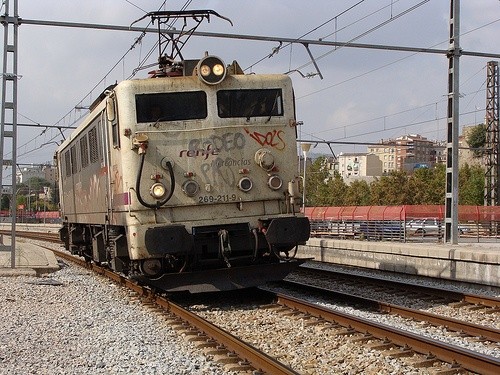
[51,51,314,294]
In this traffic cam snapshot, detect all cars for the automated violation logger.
[401,216,461,236]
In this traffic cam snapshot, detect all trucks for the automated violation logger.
[361,220,401,240]
